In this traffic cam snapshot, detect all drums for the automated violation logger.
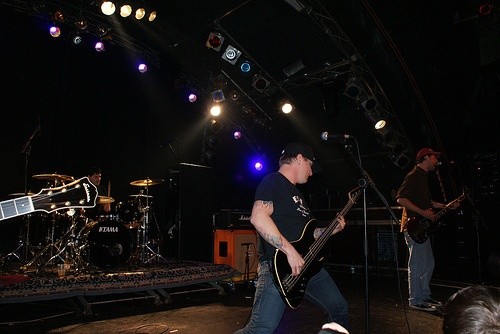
[84,195,117,218]
[116,199,145,229]
[75,220,137,274]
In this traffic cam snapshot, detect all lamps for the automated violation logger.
[344,80,414,170]
[49,0,297,162]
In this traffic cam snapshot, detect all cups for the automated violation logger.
[57,264,66,278]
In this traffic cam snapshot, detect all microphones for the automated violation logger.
[321,131,354,141]
[241,242,253,246]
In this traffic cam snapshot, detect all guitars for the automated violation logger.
[401,188,471,245]
[272,186,365,309]
[0,176,99,221]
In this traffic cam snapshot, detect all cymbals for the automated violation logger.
[32,173,75,181]
[130,194,154,198]
[7,193,34,195]
[130,178,167,186]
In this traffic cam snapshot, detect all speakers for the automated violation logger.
[213,229,259,282]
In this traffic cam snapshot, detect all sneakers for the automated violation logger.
[410,298,444,310]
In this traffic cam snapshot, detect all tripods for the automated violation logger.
[0,182,167,286]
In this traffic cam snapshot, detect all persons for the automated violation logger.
[440,285,500,334]
[396,148,460,310]
[231,141,349,334]
[77,167,109,219]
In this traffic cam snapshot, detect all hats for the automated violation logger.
[280,141,316,162]
[416,148,442,162]
